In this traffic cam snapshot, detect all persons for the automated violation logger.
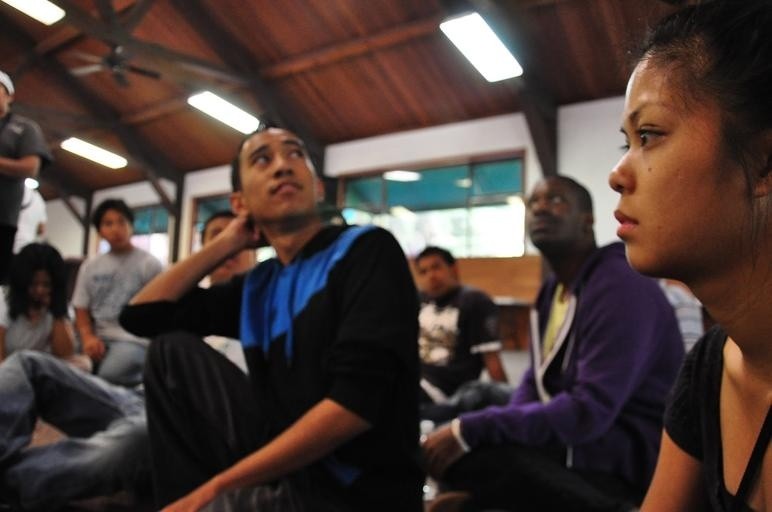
[0,210,258,512]
[0,65,58,291]
[605,1,772,511]
[70,196,165,393]
[112,123,430,512]
[420,171,691,511]
[12,183,50,257]
[0,240,95,379]
[415,243,515,431]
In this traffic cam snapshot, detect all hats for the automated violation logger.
[0,70,15,97]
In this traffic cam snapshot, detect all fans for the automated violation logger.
[52,0,163,93]
